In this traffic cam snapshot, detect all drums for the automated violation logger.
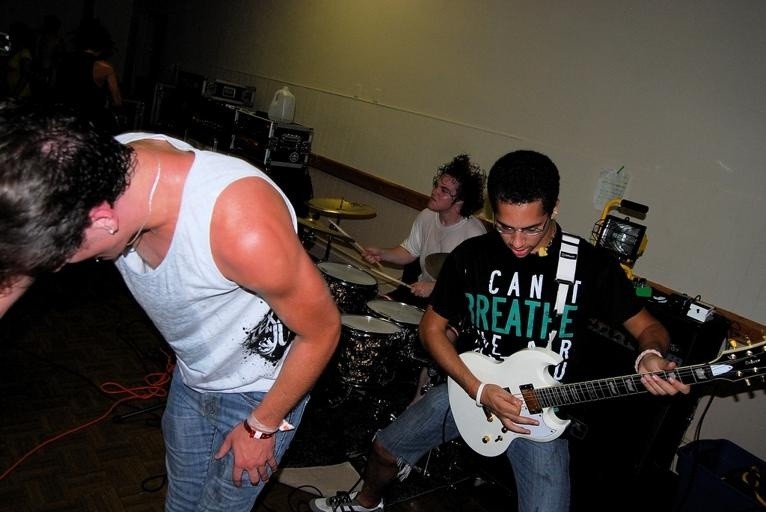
[362,296,425,367]
[336,312,401,406]
[314,260,379,317]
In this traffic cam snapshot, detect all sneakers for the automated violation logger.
[308,489,386,511]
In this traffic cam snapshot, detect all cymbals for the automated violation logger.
[304,192,375,217]
[428,251,452,275]
[294,216,357,245]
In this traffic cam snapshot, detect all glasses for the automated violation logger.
[494,213,550,236]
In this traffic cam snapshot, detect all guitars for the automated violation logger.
[446,341,766,460]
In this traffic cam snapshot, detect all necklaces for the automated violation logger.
[125,138,161,249]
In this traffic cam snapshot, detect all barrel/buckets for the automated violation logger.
[267,85,297,124]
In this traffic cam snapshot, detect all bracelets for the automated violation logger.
[634,347,663,370]
[243,419,279,440]
[245,413,279,436]
[474,382,489,405]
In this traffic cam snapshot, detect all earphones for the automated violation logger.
[93,218,115,233]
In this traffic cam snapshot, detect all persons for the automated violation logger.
[307,148,692,512]
[0,109,341,512]
[0,6,135,129]
[360,151,489,407]
